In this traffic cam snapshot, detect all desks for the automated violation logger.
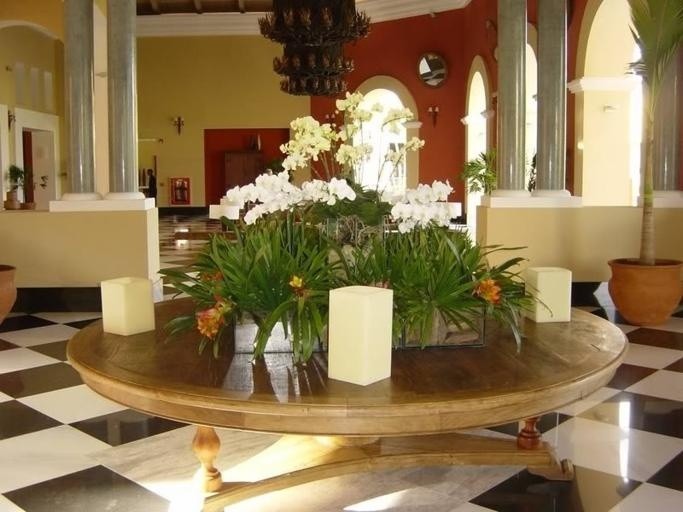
[61,297,632,512]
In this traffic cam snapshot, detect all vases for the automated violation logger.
[232,298,501,357]
[0,264,18,324]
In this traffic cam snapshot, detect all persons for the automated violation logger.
[147,168,157,197]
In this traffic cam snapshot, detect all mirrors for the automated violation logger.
[415,51,448,91]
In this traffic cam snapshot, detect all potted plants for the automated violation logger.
[605,0,681,327]
[4,161,24,211]
[21,172,49,209]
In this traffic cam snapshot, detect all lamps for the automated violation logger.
[245,0,373,96]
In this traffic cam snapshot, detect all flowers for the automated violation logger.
[147,87,554,366]
